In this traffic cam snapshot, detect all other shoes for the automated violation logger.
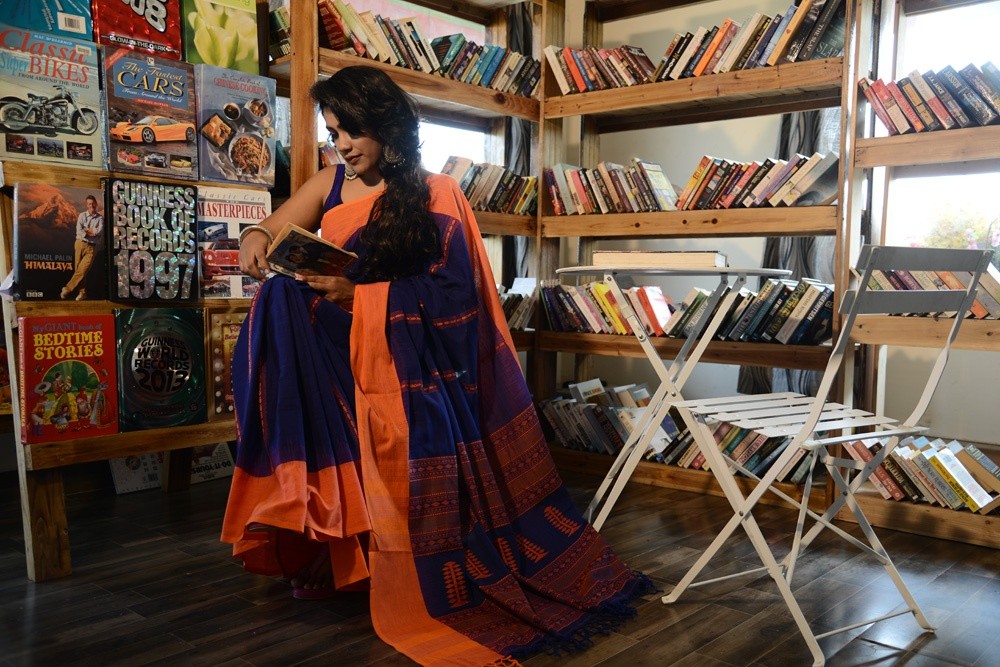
[291,557,335,600]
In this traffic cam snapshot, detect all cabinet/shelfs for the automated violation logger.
[255,0,542,394]
[541,0,878,522]
[832,0,1000,549]
[0,0,271,581]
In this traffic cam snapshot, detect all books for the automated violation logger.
[545,151,840,217]
[849,261,1000,320]
[440,156,538,216]
[257,222,359,280]
[276,140,345,200]
[841,435,1000,515]
[538,378,829,485]
[496,277,834,345]
[858,62,1000,136]
[0,0,275,494]
[593,249,727,271]
[269,0,542,97]
[543,0,856,96]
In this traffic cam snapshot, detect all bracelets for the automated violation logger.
[238,224,275,246]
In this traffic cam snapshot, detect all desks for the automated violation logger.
[554,264,794,529]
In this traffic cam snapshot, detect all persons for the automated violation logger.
[219,66,656,667]
[58,195,105,300]
[285,242,348,275]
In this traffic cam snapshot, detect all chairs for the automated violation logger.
[661,244,995,667]
